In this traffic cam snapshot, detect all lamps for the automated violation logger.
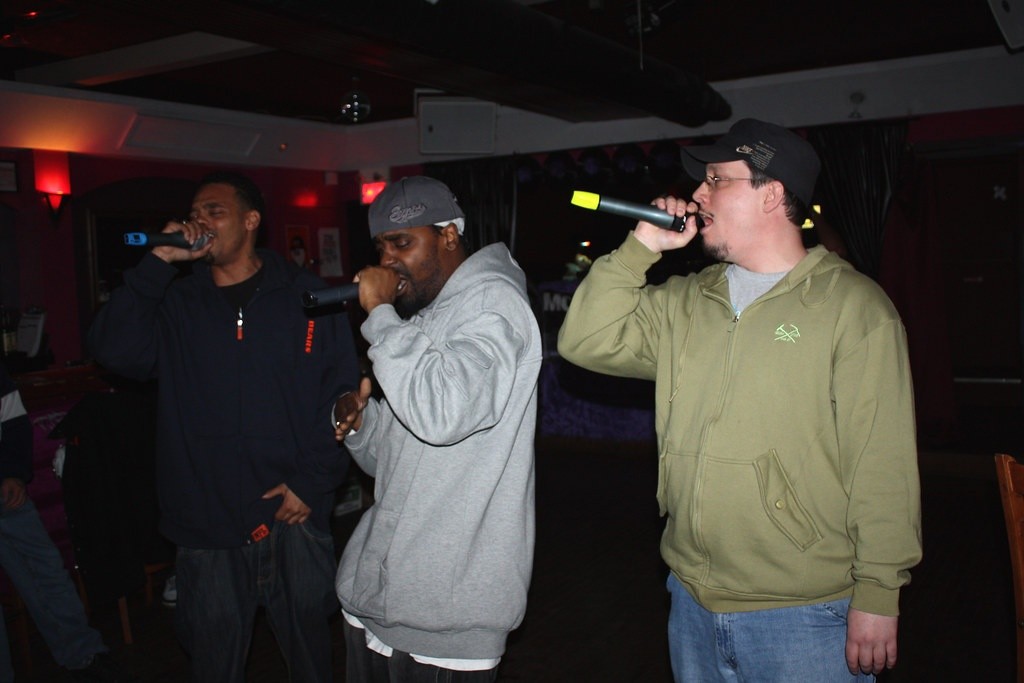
[357,162,389,205]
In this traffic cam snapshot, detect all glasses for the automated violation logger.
[704,176,752,192]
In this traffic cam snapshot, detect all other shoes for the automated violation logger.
[71,653,143,683]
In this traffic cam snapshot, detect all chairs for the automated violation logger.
[994,453,1024,683]
[0,463,92,674]
[48,384,177,644]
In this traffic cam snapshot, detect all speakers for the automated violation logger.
[986,0,1024,52]
[416,97,498,154]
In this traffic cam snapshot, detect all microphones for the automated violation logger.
[570,190,687,233]
[301,282,360,307]
[125,231,208,251]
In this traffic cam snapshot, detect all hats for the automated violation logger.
[680,118,821,207]
[368,176,465,240]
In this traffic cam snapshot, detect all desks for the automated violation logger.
[13,360,116,466]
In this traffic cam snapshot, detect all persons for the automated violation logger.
[554,121,928,681]
[0,300,127,681]
[82,167,370,683]
[322,172,546,683]
